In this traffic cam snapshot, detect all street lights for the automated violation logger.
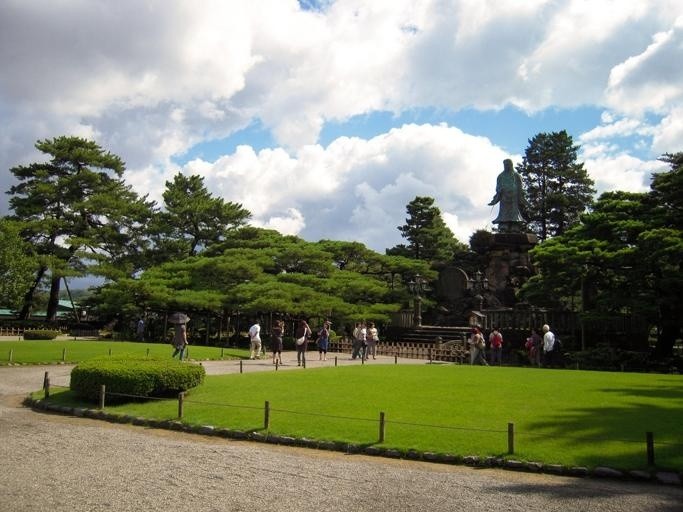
[407,273,428,328]
[465,269,490,311]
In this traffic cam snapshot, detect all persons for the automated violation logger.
[540,324,556,368]
[134,316,145,342]
[169,323,188,361]
[468,324,489,366]
[486,325,504,366]
[294,319,312,367]
[347,321,367,361]
[269,320,284,365]
[365,322,378,360]
[486,158,531,232]
[315,323,329,361]
[524,327,543,367]
[247,319,262,360]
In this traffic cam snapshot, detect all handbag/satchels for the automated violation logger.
[297,336,305,346]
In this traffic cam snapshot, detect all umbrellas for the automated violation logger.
[166,311,189,325]
